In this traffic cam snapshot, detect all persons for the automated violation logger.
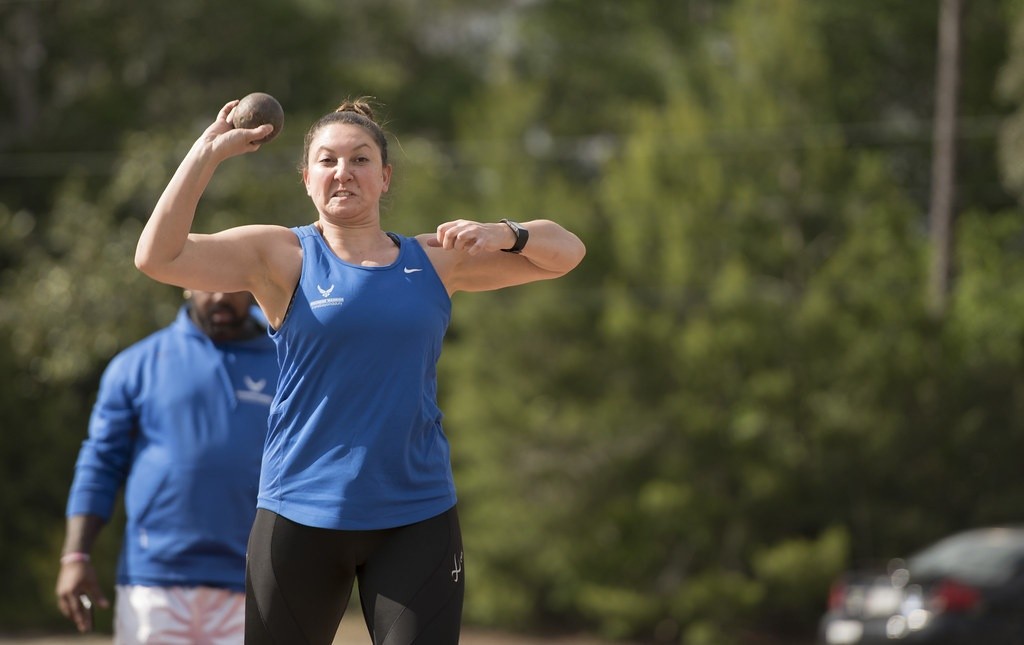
[132,95,589,645]
[52,284,279,645]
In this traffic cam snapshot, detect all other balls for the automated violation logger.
[232,91,285,146]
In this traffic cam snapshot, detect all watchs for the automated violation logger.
[497,218,529,254]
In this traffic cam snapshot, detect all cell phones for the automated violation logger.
[80,594,94,631]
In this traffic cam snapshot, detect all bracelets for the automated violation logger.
[58,553,91,564]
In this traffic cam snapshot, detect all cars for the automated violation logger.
[814,521,1024,645]
